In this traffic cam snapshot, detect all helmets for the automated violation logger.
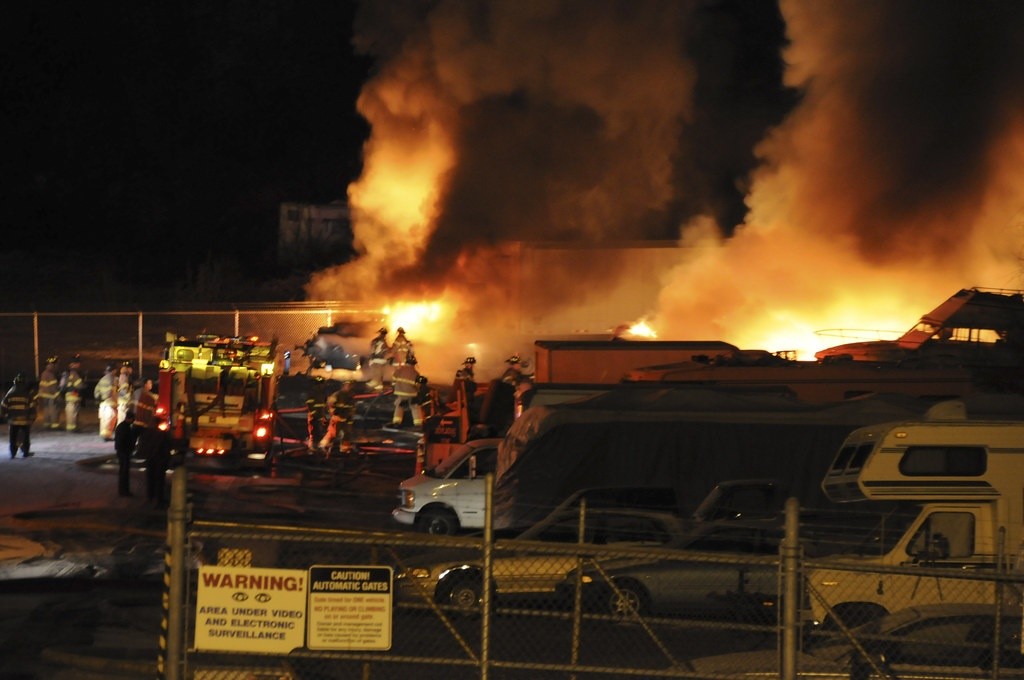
[466,357,476,364]
[106,364,116,371]
[407,357,417,364]
[509,356,519,363]
[13,375,24,384]
[47,355,58,363]
[415,376,428,384]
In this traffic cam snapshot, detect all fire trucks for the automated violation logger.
[153,326,279,475]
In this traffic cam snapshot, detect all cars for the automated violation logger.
[391,436,504,538]
[393,474,887,626]
[659,601,1024,679]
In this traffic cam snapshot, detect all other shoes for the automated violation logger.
[12,455,15,458]
[24,452,34,456]
[122,491,134,497]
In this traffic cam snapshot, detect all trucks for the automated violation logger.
[534,337,770,384]
[813,286,1024,366]
[793,418,1024,630]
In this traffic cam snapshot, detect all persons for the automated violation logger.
[0,326,535,502]
